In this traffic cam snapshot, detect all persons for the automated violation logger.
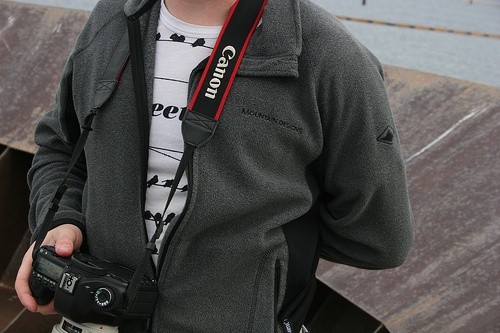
[14,0,413,333]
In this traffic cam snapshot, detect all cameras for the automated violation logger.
[29,245,158,333]
